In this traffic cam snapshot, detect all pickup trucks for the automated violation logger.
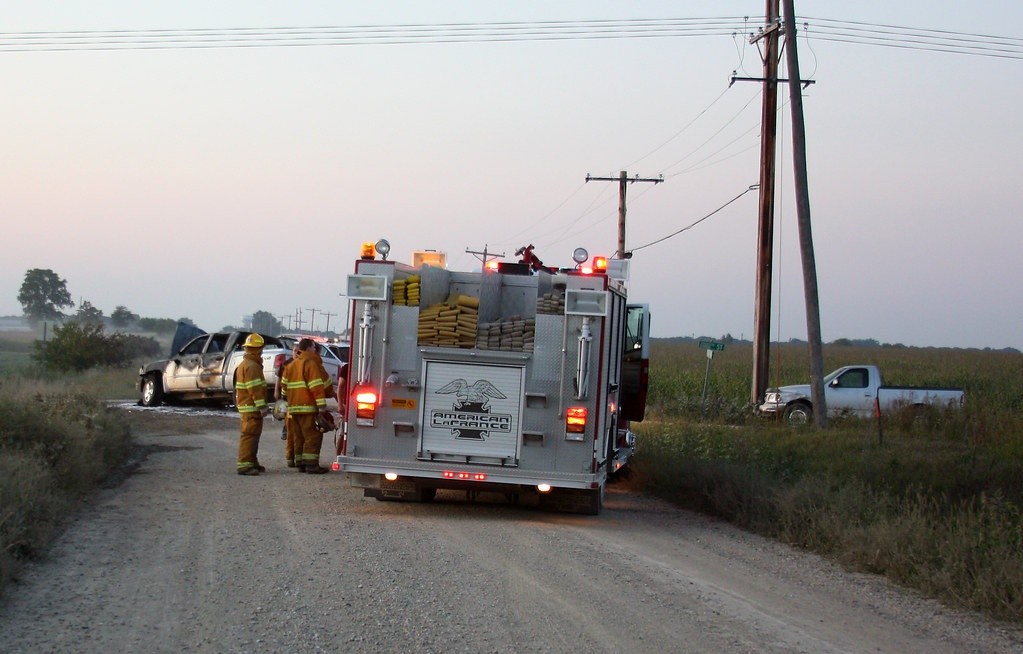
[758,366,965,429]
[136,331,350,406]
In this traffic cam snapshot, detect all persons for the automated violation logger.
[236,334,269,476]
[273,339,335,474]
[336,363,349,461]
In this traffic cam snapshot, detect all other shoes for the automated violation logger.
[306,465,329,474]
[254,464,265,472]
[288,464,300,468]
[298,465,306,473]
[281,426,287,440]
[238,467,260,476]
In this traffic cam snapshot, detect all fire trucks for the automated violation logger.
[332,237,650,513]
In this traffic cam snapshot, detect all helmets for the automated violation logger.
[242,333,264,347]
[313,410,335,433]
[272,399,287,421]
